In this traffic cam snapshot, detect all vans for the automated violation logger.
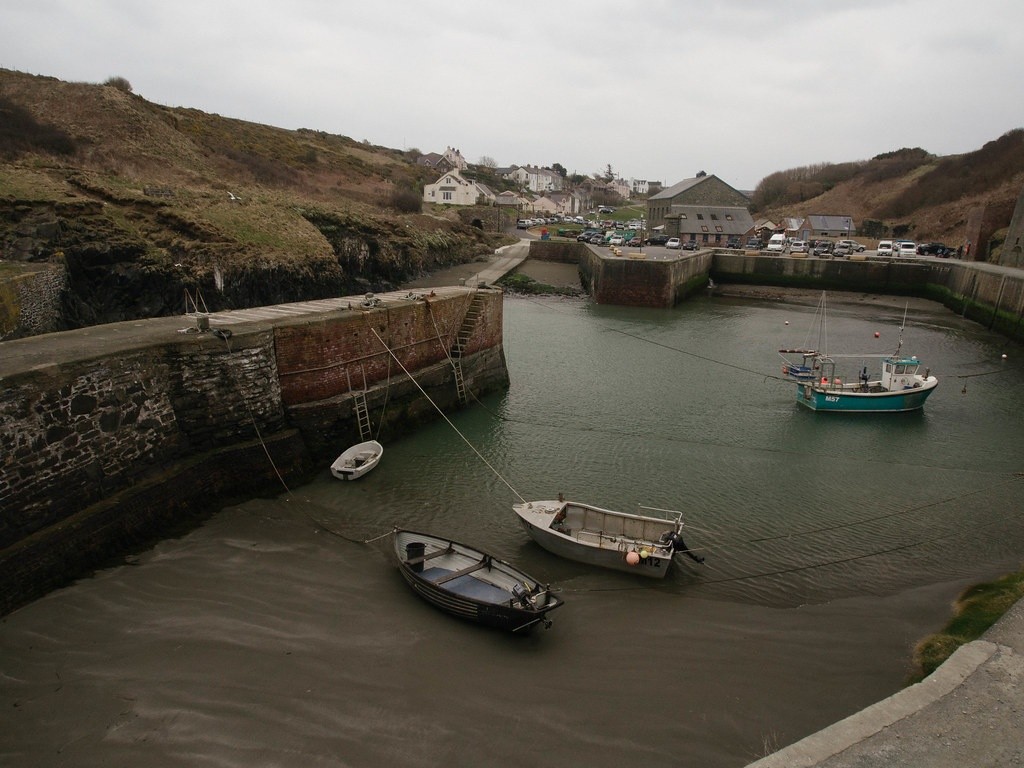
[768,234,787,252]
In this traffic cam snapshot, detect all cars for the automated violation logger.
[665,238,682,249]
[517,205,669,247]
[745,238,763,249]
[876,241,892,256]
[789,241,810,255]
[682,240,700,250]
[918,242,956,256]
[726,237,741,249]
[832,245,854,255]
[835,239,867,252]
[813,242,833,255]
[892,240,917,257]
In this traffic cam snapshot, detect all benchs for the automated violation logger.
[431,560,491,585]
[404,547,455,564]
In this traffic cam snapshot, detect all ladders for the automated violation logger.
[453,361,467,405]
[354,392,372,442]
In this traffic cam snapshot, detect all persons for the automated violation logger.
[936,243,971,260]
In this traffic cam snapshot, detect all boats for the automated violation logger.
[510,493,684,578]
[392,526,566,629]
[330,440,383,481]
[781,351,939,413]
